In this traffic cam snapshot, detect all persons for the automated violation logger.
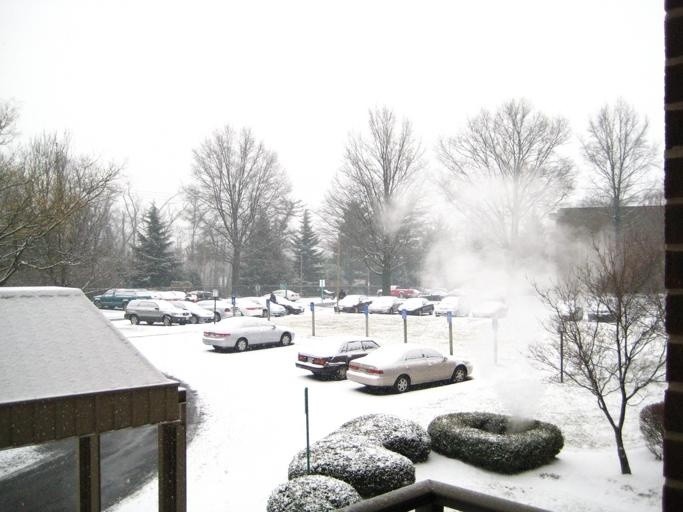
[270,292,276,303]
[339,289,345,299]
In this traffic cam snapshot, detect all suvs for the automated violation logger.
[124,299,193,326]
[92,288,188,310]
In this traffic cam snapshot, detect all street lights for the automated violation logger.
[291,253,303,295]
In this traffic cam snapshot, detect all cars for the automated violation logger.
[197,300,242,322]
[397,297,434,316]
[169,301,215,325]
[293,339,379,382]
[334,295,372,313]
[221,294,305,317]
[345,346,473,392]
[200,315,296,354]
[472,300,509,318]
[272,289,300,302]
[367,296,403,314]
[434,296,471,318]
